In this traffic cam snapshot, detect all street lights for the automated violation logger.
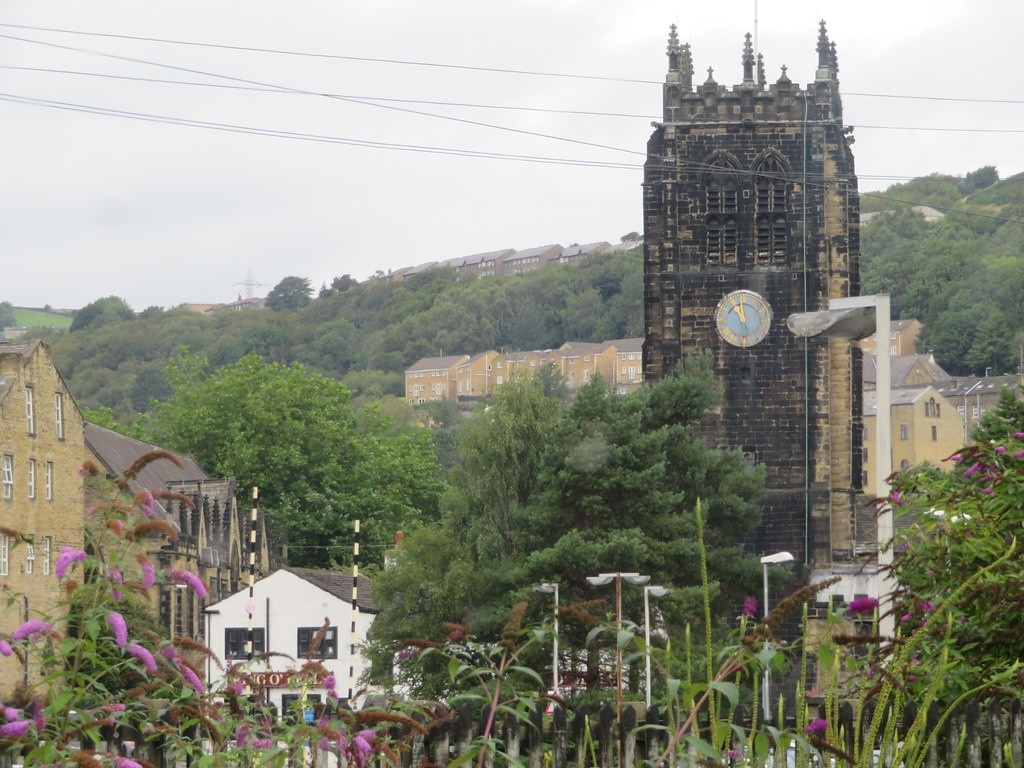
[540,583,559,693]
[585,572,651,768]
[644,585,667,711]
[760,550,795,722]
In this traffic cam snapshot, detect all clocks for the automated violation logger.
[714,290,773,348]
